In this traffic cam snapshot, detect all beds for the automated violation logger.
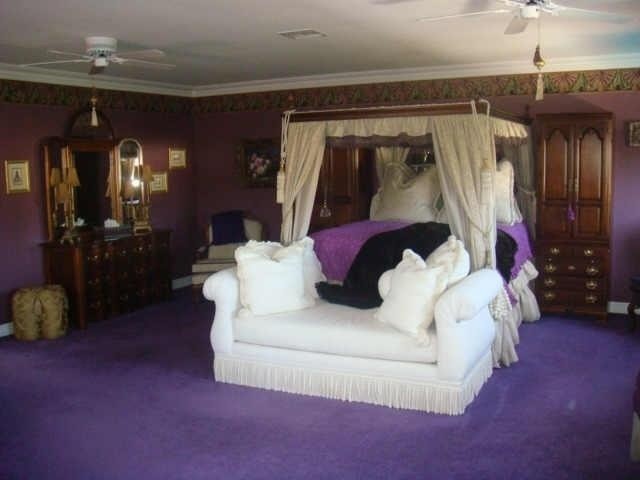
[306,162,537,367]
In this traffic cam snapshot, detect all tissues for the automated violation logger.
[104,218,132,238]
[59,218,89,232]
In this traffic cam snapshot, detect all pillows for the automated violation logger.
[234,236,325,314]
[370,158,522,226]
[375,236,471,342]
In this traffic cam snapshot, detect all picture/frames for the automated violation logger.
[145,146,186,196]
[240,139,282,188]
[627,119,640,148]
[4,159,32,195]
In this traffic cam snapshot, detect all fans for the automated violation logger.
[14,34,177,70]
[417,0,632,35]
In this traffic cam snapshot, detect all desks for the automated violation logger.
[37,228,173,331]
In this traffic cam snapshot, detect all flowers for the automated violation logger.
[247,153,270,176]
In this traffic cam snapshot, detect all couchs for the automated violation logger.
[201,267,503,415]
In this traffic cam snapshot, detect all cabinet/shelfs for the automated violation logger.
[531,105,614,326]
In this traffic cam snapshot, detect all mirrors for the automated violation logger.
[41,136,150,244]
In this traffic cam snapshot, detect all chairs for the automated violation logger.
[191,209,267,298]
